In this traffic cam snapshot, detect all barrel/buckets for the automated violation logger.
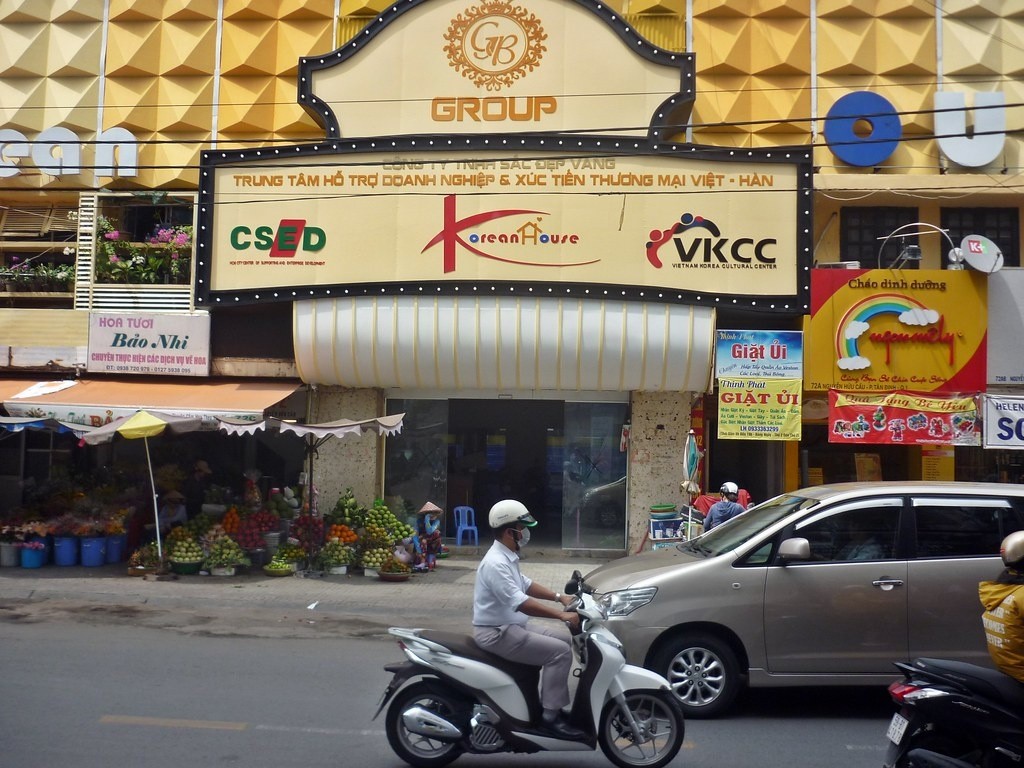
[0,533,127,568]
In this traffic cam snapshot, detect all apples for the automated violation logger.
[269,543,352,569]
[167,512,244,564]
[361,506,416,567]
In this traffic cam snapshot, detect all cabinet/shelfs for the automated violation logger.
[0,241,192,299]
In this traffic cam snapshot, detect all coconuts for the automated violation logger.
[324,496,362,525]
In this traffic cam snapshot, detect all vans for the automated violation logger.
[577,480,1024,719]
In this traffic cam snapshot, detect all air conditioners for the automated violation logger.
[816,261,861,269]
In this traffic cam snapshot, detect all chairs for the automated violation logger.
[453,505,479,547]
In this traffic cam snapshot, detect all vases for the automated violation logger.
[5,283,16,292]
[67,282,74,292]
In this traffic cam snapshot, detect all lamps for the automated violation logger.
[887,244,922,270]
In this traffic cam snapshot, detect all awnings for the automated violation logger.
[0,371,306,432]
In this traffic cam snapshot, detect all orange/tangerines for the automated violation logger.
[326,523,358,542]
[220,507,241,532]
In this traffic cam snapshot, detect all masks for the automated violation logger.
[513,528,530,548]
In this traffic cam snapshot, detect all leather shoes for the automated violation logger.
[537,714,585,741]
[557,707,572,718]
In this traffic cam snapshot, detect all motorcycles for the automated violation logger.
[882,656,1024,768]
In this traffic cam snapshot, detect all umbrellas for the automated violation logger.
[213,413,406,570]
[81,408,202,560]
[681,429,704,541]
[0,415,100,440]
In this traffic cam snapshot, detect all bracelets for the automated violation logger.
[555,593,560,602]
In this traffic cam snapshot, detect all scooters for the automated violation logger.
[371,571,686,768]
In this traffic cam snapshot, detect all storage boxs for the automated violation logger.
[266,546,278,563]
[328,565,348,575]
[287,537,299,546]
[128,567,160,577]
[648,516,683,540]
[263,530,285,546]
[435,433,464,459]
[379,543,414,554]
[542,430,568,487]
[486,434,512,472]
[291,560,306,572]
[249,548,266,570]
[685,524,704,541]
[650,540,683,551]
[363,569,380,576]
[210,566,237,577]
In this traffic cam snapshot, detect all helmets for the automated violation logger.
[489,499,536,528]
[720,482,739,495]
[1000,531,1024,566]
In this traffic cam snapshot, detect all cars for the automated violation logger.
[572,477,627,527]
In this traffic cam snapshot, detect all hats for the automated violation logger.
[195,461,211,474]
[418,502,443,515]
[163,489,185,499]
[510,521,537,527]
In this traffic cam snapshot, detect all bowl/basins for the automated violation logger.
[378,571,411,581]
[264,566,291,576]
[168,560,204,575]
[649,504,678,519]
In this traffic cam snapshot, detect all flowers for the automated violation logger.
[0,485,131,550]
[0,210,193,284]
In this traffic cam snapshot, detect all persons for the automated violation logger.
[810,509,888,561]
[472,500,587,739]
[416,501,444,572]
[978,531,1024,685]
[183,459,211,519]
[704,482,746,534]
